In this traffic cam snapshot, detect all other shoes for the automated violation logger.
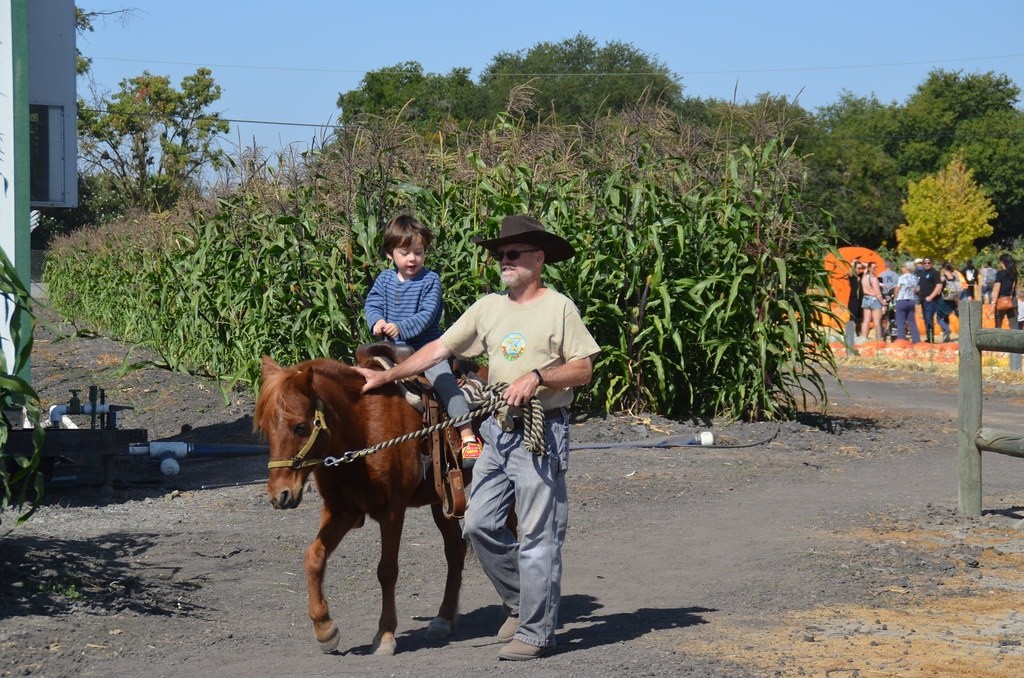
[497,615,520,643]
[497,638,553,661]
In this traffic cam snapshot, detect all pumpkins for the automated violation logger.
[812,305,1011,335]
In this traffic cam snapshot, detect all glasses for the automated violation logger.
[924,263,930,264]
[492,249,544,261]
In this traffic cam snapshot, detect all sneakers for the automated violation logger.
[461,436,483,473]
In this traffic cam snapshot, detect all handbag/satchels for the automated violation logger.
[996,296,1013,312]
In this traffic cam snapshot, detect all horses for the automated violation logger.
[252,327,489,653]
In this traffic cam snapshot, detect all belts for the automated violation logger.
[490,406,571,433]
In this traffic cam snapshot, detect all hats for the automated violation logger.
[923,257,931,262]
[471,214,575,264]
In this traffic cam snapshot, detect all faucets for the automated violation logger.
[66,388,134,414]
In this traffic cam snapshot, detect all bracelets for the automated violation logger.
[532,368,543,387]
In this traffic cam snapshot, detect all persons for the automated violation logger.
[347,214,601,661]
[365,213,482,461]
[847,252,1024,343]
